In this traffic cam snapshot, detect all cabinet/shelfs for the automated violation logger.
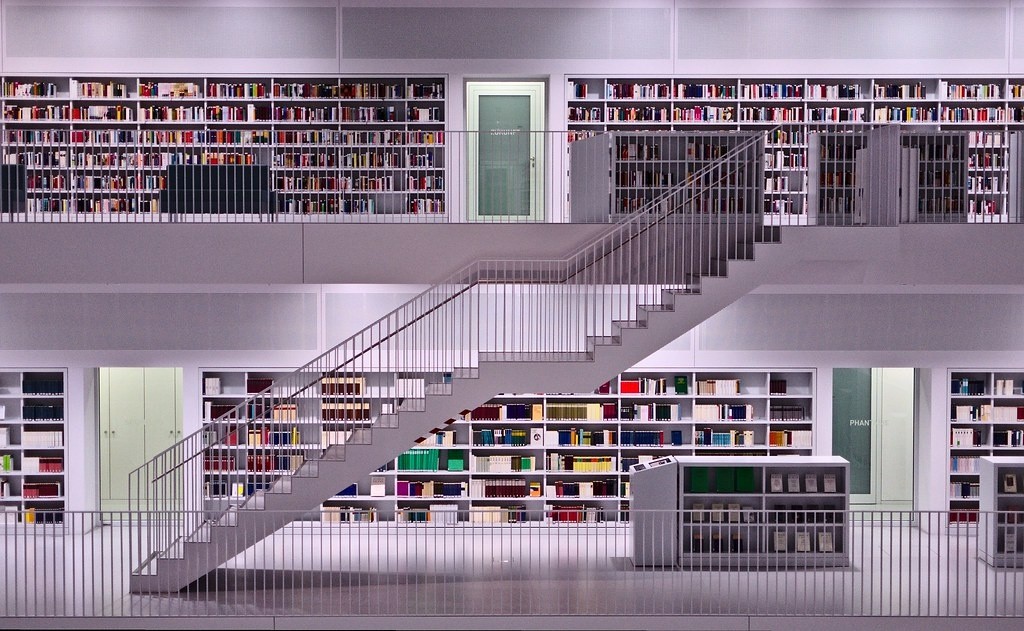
[624,452,853,560]
[0,365,71,535]
[191,360,820,535]
[943,366,1023,532]
[976,454,1024,571]
[563,73,1024,223]
[3,72,451,223]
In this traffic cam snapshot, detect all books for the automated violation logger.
[568,77,1022,218]
[204,370,840,554]
[0,371,61,524]
[0,74,446,215]
[949,371,1023,553]
